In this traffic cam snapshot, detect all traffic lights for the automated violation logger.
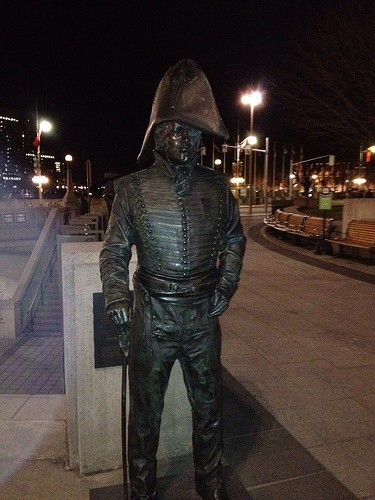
[365,150,372,164]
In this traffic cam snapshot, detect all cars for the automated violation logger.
[229,180,375,206]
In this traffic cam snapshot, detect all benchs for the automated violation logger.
[263,209,375,263]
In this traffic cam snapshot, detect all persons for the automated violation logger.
[98,56,249,500]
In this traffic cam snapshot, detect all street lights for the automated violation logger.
[236,133,258,205]
[35,119,52,205]
[240,91,263,214]
[64,155,73,193]
